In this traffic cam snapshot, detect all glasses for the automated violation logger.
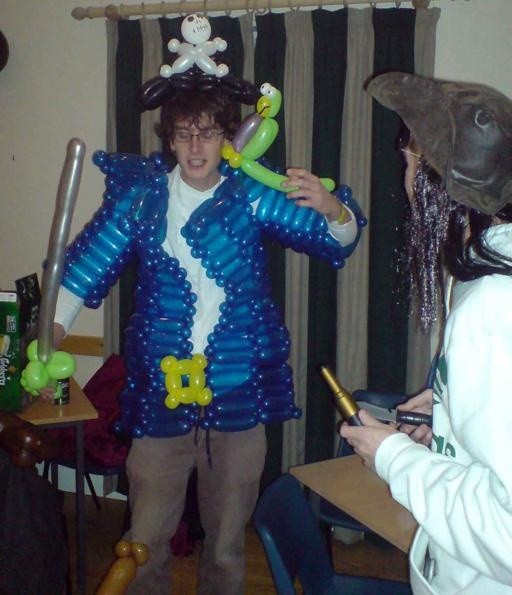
[174,131,222,143]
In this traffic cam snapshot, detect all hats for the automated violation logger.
[367,72,512,215]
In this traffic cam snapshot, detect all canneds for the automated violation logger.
[55,378,70,406]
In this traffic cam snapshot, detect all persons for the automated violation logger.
[338,74,512,595]
[38,89,366,595]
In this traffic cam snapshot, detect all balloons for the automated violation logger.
[93,539,149,595]
[140,13,257,111]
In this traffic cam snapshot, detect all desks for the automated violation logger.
[18,371,99,594]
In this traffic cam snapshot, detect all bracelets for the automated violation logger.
[335,205,345,226]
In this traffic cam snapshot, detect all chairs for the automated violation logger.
[250,388,413,595]
